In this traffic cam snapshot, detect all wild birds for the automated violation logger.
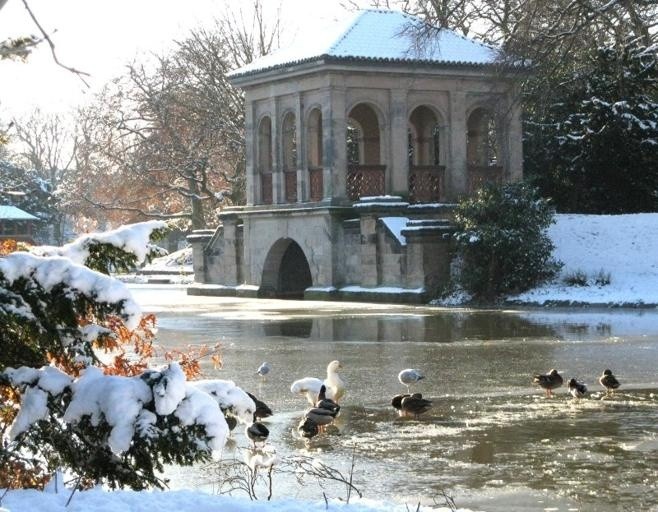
[599,368,620,396]
[255,360,270,382]
[290,376,340,443]
[254,401,273,422]
[391,392,432,420]
[566,378,591,401]
[246,417,270,449]
[397,368,426,391]
[323,359,345,405]
[533,368,563,397]
